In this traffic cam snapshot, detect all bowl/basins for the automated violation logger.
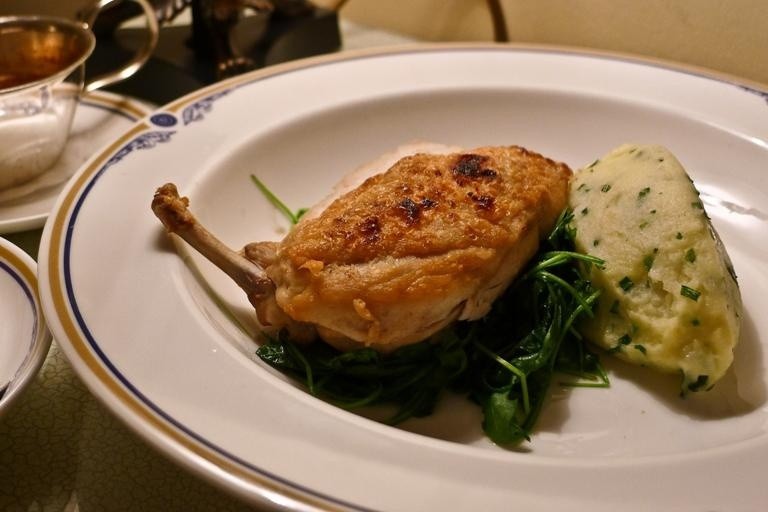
[1,239,57,416]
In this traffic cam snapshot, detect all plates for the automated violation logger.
[3,80,149,231]
[31,42,765,512]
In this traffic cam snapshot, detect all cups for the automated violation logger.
[2,2,154,184]
